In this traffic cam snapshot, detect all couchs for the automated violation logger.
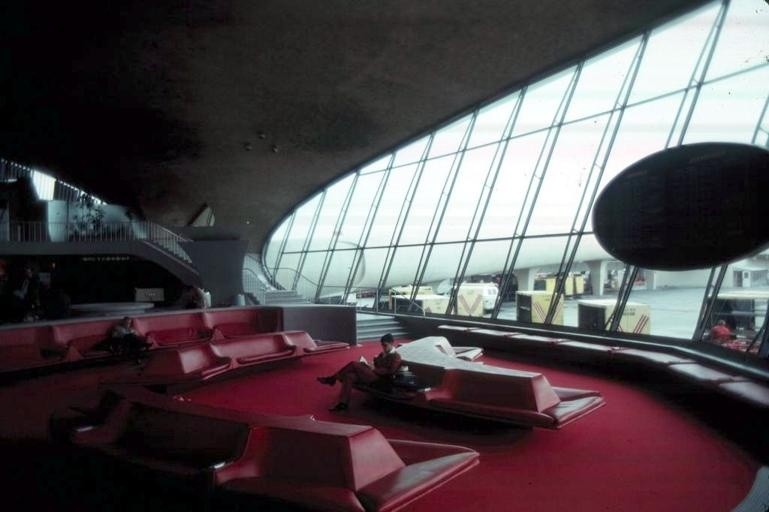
[351,335,608,429]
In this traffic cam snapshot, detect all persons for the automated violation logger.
[703,318,732,345]
[316,331,406,413]
[109,315,148,364]
[21,267,42,316]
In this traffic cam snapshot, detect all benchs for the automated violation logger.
[609,349,695,393]
[132,307,286,360]
[667,363,750,427]
[712,379,768,465]
[135,329,349,396]
[508,334,570,365]
[1,310,158,374]
[438,324,482,348]
[466,329,525,352]
[49,378,480,512]
[556,340,629,371]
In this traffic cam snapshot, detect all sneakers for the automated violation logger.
[329,402,346,411]
[318,375,336,387]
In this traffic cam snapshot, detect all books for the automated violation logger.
[359,355,372,368]
